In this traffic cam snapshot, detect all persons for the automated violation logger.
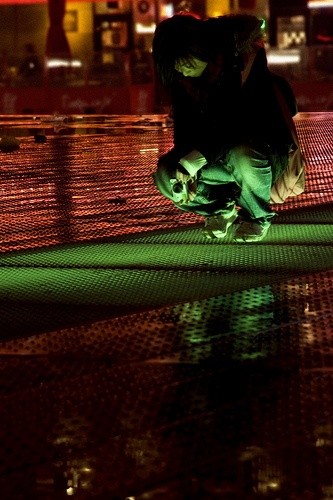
[16,44,45,87]
[173,1,201,21]
[150,13,304,243]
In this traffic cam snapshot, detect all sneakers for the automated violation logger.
[232,219,272,242]
[201,206,238,239]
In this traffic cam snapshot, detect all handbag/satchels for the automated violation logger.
[268,144,307,205]
